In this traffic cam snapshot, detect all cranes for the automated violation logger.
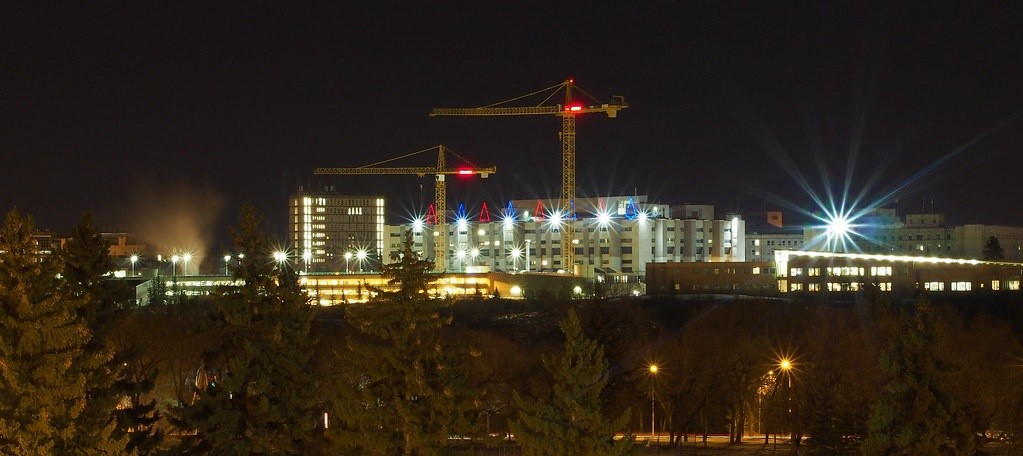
[313,144,497,272]
[427,80,630,273]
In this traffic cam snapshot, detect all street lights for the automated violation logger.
[172,255,179,276]
[345,253,351,273]
[304,252,311,275]
[649,365,658,437]
[471,247,479,267]
[184,254,192,276]
[782,361,791,416]
[503,217,512,252]
[131,255,138,277]
[456,219,467,252]
[224,256,230,274]
[412,221,422,247]
[458,251,464,274]
[512,249,521,274]
[237,253,244,264]
[358,251,366,272]
[278,251,288,274]
